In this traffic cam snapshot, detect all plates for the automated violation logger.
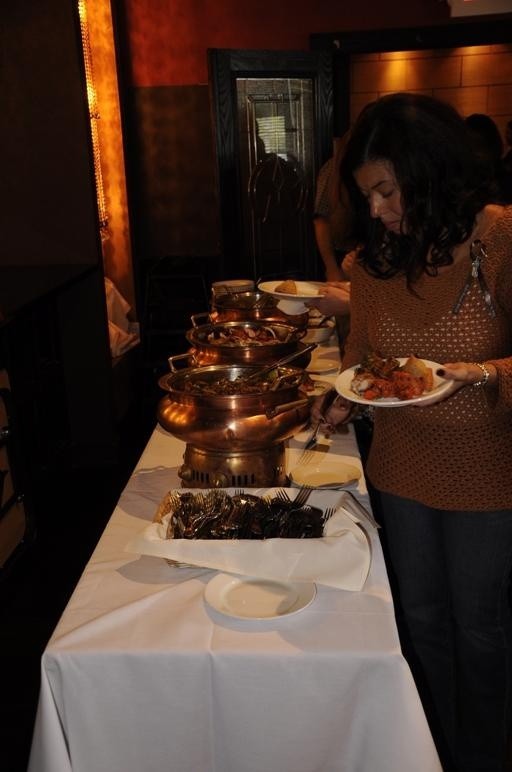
[204,571,317,621]
[333,357,456,410]
[304,357,340,397]
[209,278,257,312]
[256,278,326,302]
[288,459,361,490]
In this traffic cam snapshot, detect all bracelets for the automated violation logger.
[471,362,490,392]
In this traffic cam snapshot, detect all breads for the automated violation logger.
[275,279,297,294]
[404,354,434,392]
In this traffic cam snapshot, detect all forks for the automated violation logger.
[168,483,336,540]
[300,388,339,464]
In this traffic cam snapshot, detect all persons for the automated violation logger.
[465,111,504,194]
[304,88,512,768]
[497,121,512,207]
[307,245,366,325]
[311,107,364,281]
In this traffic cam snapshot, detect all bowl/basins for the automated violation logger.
[298,318,336,344]
